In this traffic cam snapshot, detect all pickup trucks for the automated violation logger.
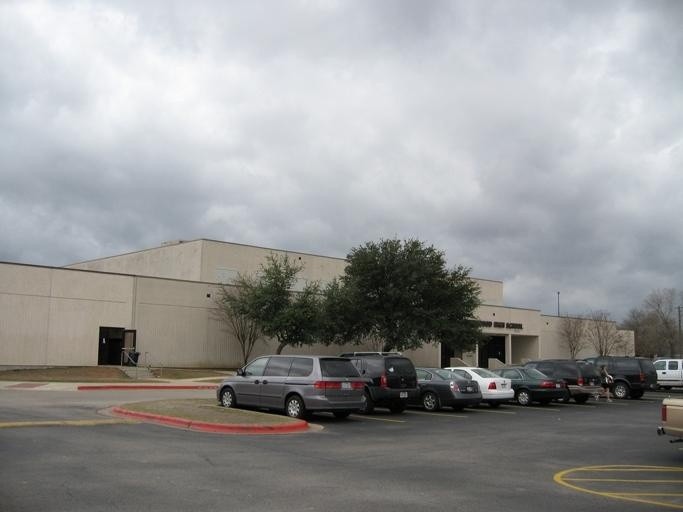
[652,358,683,389]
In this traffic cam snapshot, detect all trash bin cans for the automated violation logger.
[128,351,139,366]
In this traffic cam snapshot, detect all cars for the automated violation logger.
[658,392,682,448]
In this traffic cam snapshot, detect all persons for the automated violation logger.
[595,364,615,401]
